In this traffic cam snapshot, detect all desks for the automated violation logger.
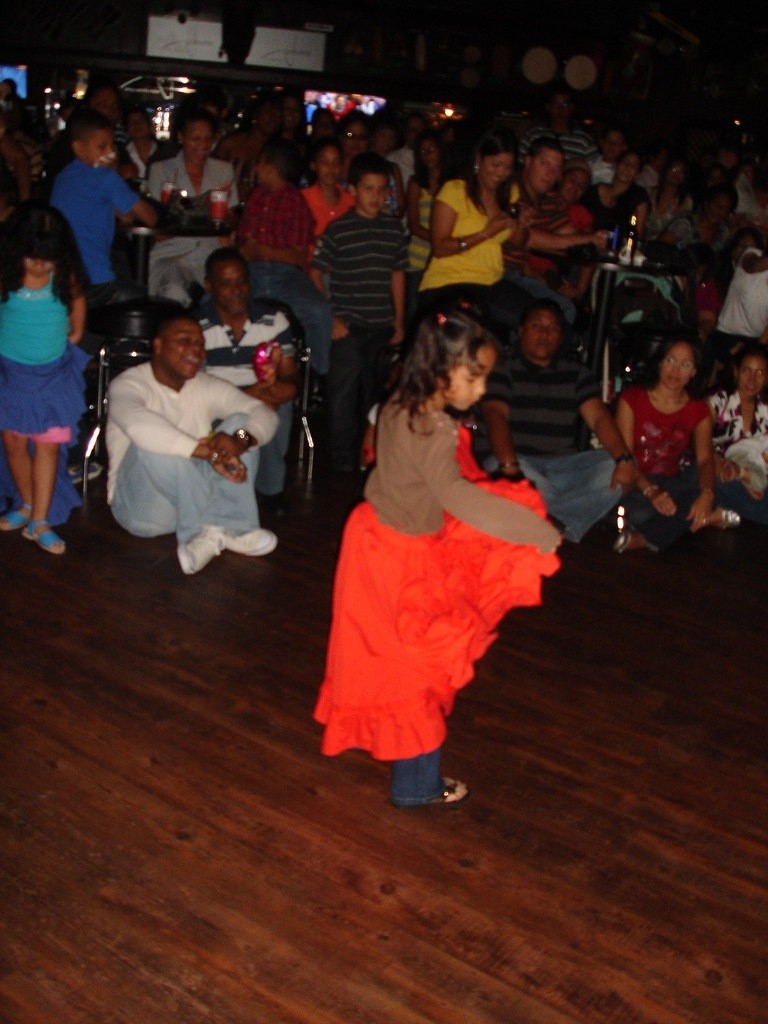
[122,223,238,297]
[585,255,693,394]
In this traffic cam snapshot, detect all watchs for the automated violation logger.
[611,451,634,465]
[234,427,253,447]
[456,238,468,251]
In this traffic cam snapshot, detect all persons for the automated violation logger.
[315,311,565,808]
[0,78,768,576]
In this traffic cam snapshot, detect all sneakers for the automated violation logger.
[220,525,278,557]
[176,528,226,574]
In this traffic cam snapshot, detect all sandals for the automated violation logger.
[21,519,65,554]
[0,502,34,531]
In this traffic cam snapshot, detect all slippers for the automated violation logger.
[393,776,474,807]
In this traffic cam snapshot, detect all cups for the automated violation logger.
[161,181,174,204]
[209,189,228,223]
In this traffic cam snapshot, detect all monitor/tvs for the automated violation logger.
[301,89,388,139]
[0,66,28,99]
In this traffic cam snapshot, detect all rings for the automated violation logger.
[702,517,707,525]
[238,463,246,470]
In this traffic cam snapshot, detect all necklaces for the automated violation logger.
[650,388,688,407]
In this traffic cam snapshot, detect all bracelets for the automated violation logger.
[209,448,220,466]
[497,460,520,469]
[642,484,660,496]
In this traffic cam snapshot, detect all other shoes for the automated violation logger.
[703,507,742,531]
[614,531,633,554]
[66,463,102,483]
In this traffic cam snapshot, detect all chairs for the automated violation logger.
[79,296,315,500]
